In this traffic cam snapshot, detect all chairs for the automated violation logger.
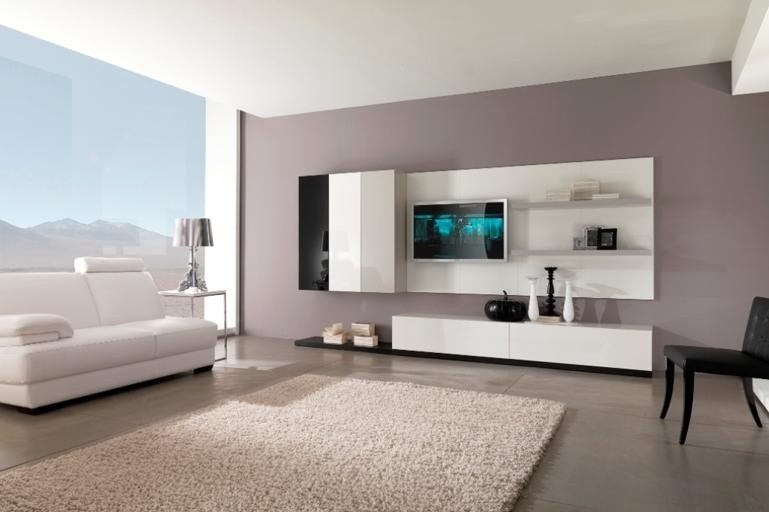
[659,297,769,445]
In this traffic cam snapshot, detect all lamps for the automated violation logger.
[173,217,213,293]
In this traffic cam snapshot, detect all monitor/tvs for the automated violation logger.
[407,198,508,264]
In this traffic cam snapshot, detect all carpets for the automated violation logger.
[0,375,567,512]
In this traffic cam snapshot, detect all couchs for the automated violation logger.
[0,257,217,415]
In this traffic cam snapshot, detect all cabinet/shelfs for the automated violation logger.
[294,155,655,300]
[392,312,508,365]
[509,322,652,376]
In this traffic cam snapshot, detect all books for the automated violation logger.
[544,178,619,201]
